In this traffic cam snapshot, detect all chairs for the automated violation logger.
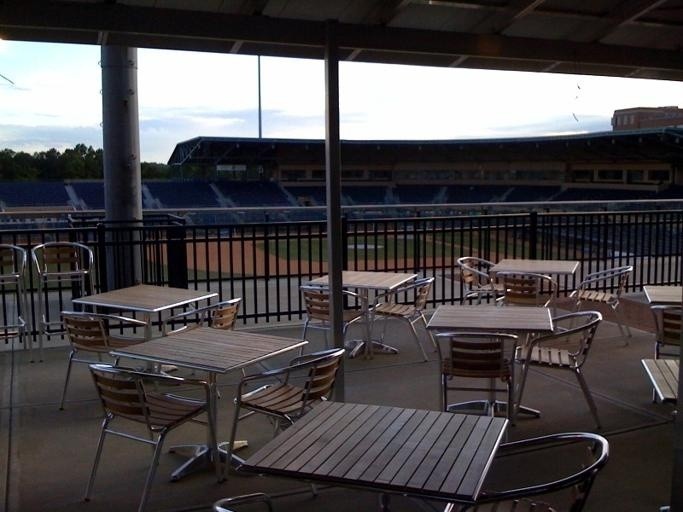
[457,257,503,305]
[215,493,274,512]
[224,348,345,478]
[169,296,244,397]
[0,245,28,352]
[59,311,144,411]
[568,267,632,352]
[438,432,611,512]
[434,331,517,433]
[513,311,602,431]
[502,272,560,344]
[368,276,437,363]
[33,241,97,363]
[650,305,683,402]
[84,366,224,512]
[301,285,370,360]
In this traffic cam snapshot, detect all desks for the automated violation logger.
[108,326,308,481]
[73,285,218,382]
[489,259,579,294]
[312,272,418,357]
[643,360,682,406]
[645,286,683,320]
[426,306,553,419]
